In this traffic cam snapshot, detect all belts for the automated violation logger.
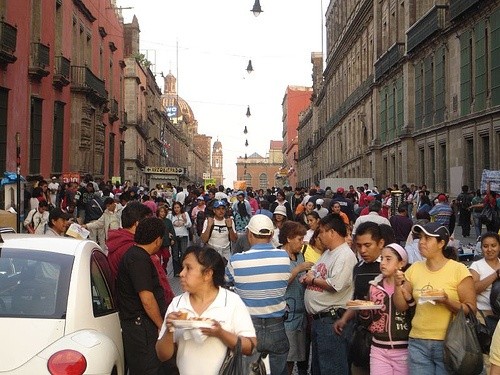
[313,311,337,321]
[177,236,187,238]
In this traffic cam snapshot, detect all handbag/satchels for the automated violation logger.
[218,336,266,375]
[490,269,500,316]
[480,204,500,227]
[466,303,499,355]
[346,328,372,375]
[444,305,483,375]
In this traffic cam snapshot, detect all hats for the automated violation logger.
[274,205,287,217]
[316,199,325,205]
[245,214,273,235]
[52,177,57,180]
[412,223,450,237]
[49,208,70,219]
[196,196,204,201]
[438,195,446,200]
[213,200,226,207]
[337,187,344,193]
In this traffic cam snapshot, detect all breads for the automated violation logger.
[347,301,364,306]
[176,312,188,320]
[427,290,446,296]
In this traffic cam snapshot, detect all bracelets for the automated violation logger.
[312,277,315,285]
[227,227,233,229]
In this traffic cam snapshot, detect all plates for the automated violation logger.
[418,294,445,301]
[172,319,216,329]
[350,305,383,310]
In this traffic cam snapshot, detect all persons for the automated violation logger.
[24,175,500,375]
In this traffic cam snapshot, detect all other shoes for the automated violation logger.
[174,274,179,277]
[463,233,470,237]
[477,236,481,242]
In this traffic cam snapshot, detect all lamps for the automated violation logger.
[243,0,263,176]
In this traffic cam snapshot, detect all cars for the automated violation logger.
[0,233,125,375]
